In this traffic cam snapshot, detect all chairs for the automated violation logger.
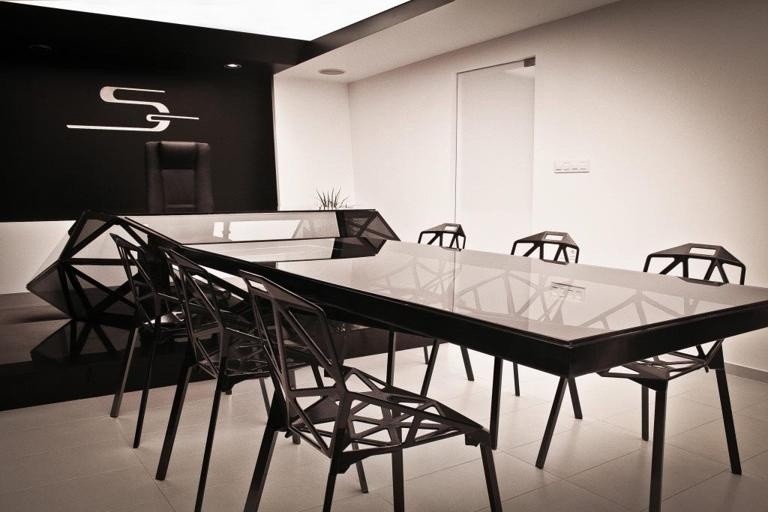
[146,141,216,215]
